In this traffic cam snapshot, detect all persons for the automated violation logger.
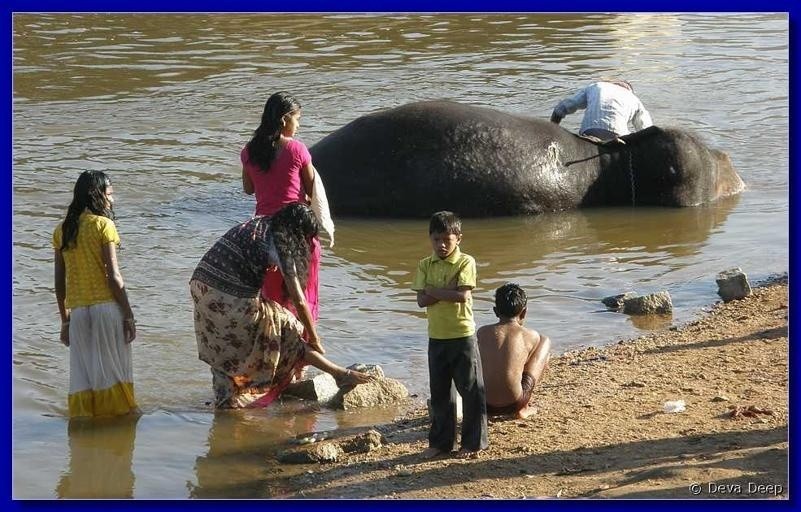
[410,210,490,460]
[189,201,375,410]
[240,91,335,382]
[52,169,143,419]
[550,78,653,144]
[477,283,552,420]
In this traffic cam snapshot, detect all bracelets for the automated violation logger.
[309,340,320,346]
[125,318,137,325]
[61,320,70,326]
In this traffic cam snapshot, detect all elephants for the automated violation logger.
[309,99,746,220]
